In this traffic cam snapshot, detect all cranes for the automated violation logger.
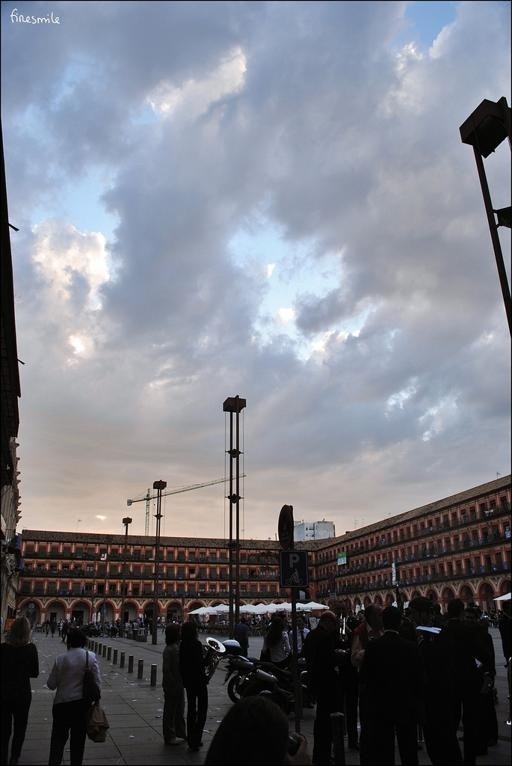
[127,472,248,537]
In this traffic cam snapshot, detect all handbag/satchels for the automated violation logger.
[81,651,102,709]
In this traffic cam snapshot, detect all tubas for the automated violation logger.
[203,637,226,683]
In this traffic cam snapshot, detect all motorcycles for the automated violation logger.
[222,639,310,713]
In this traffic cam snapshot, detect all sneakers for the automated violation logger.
[166,736,199,752]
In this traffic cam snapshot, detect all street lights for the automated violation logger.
[456,94,510,330]
[221,395,248,635]
[151,479,168,601]
[121,515,131,630]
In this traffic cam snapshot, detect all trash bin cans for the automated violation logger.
[119,623,126,637]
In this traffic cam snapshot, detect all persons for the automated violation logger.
[0,615,39,765]
